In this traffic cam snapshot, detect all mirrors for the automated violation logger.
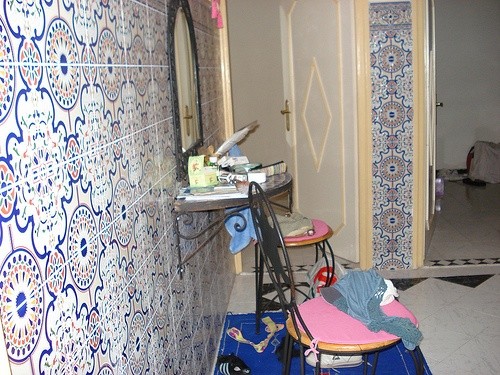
[169,0,204,181]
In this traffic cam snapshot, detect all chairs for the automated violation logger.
[247,180,426,375]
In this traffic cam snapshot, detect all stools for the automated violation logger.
[255,219,336,334]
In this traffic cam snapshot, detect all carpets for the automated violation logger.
[214,312,431,375]
